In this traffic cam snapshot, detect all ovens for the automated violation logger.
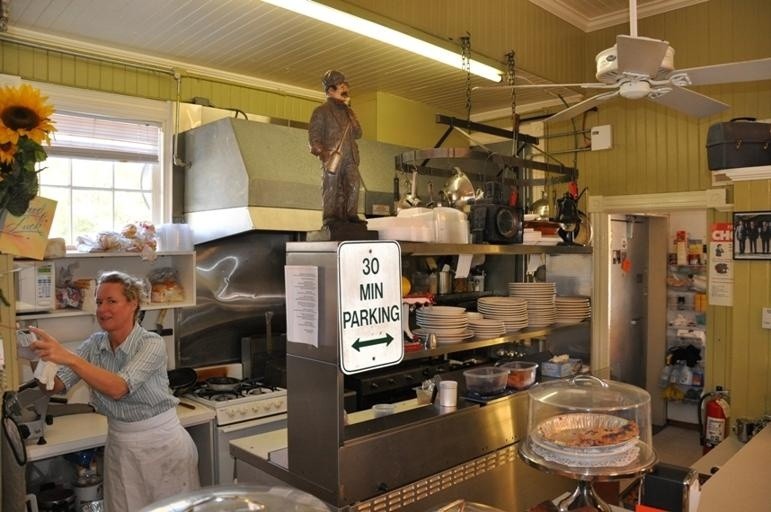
[213,412,287,485]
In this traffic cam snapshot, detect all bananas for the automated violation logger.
[662,384,683,401]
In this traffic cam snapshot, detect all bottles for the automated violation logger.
[658,360,694,388]
[675,229,689,266]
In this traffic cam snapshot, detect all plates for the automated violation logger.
[523,226,565,246]
[532,415,639,457]
[411,281,591,346]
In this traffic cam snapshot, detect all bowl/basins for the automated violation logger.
[167,367,198,395]
[502,360,538,388]
[524,220,561,235]
[463,366,509,395]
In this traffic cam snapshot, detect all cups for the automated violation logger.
[737,417,753,444]
[415,386,433,405]
[439,381,457,407]
[371,404,396,417]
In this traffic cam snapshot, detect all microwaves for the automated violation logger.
[12,260,57,314]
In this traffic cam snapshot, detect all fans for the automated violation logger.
[470,0,770,125]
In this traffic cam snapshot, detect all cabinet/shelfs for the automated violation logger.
[0,249,199,394]
[288,242,594,509]
[666,260,709,425]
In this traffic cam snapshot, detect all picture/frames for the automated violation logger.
[732,210,770,260]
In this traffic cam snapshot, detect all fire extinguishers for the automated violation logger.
[698,386,731,455]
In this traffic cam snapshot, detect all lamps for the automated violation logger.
[258,0,507,85]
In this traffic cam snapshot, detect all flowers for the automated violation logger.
[0,79,61,219]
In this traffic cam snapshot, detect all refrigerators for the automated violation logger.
[601,213,673,436]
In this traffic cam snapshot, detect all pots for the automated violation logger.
[531,190,561,220]
[204,376,250,390]
[36,474,104,511]
[400,162,478,209]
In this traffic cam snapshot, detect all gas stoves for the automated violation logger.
[181,378,287,426]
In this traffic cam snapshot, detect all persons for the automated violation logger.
[737,217,747,252]
[28,270,203,512]
[761,222,771,252]
[746,220,761,252]
[308,69,369,226]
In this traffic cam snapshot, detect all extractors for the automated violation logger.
[180,115,510,253]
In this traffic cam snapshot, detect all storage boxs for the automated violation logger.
[706,116,770,170]
